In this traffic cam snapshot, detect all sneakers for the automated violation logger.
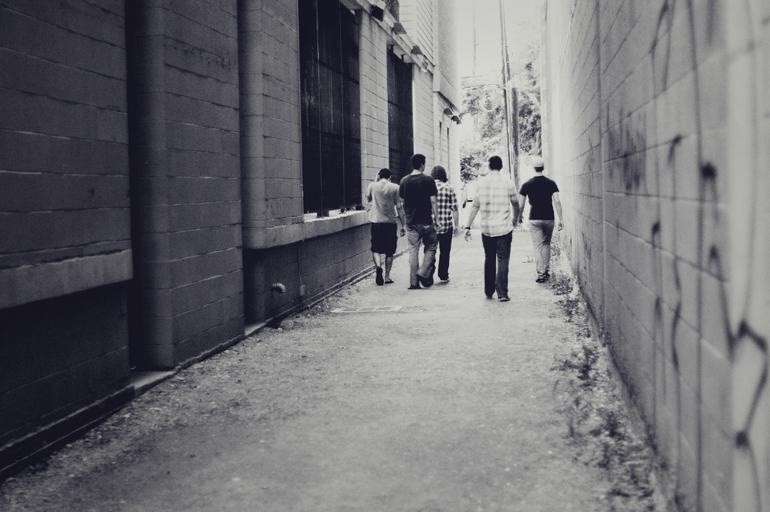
[498,296,511,302]
[485,293,493,299]
[409,272,450,289]
[535,272,550,282]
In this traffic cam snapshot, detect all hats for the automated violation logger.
[533,157,544,168]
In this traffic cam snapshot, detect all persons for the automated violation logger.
[517,157,565,283]
[365,167,407,287]
[463,155,519,302]
[399,153,443,290]
[429,165,459,282]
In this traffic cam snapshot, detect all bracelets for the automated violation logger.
[464,226,470,230]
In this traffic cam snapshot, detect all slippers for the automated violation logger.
[384,279,394,284]
[375,266,384,286]
